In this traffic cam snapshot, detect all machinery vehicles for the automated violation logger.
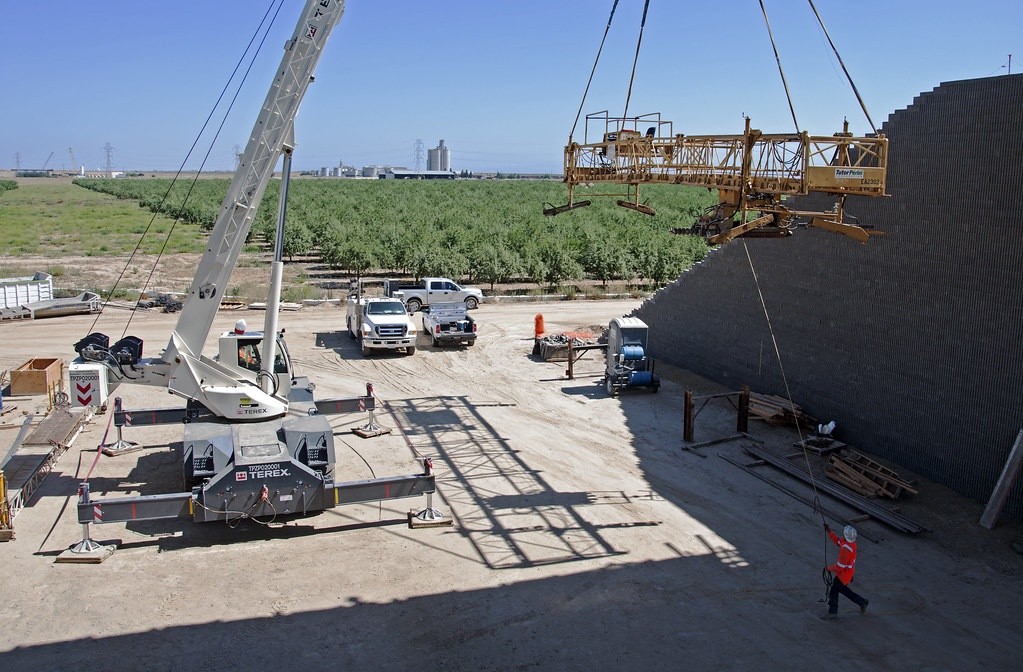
[55,0,892,563]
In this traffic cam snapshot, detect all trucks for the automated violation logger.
[346,297,417,356]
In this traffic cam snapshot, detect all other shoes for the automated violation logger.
[860,599,869,616]
[820,614,838,620]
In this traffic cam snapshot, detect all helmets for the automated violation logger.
[844,525,857,543]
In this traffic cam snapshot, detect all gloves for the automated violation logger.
[823,524,830,530]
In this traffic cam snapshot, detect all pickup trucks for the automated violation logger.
[392,277,483,312]
[422,302,478,347]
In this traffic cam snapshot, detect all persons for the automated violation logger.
[821,524,870,619]
[239,346,255,364]
